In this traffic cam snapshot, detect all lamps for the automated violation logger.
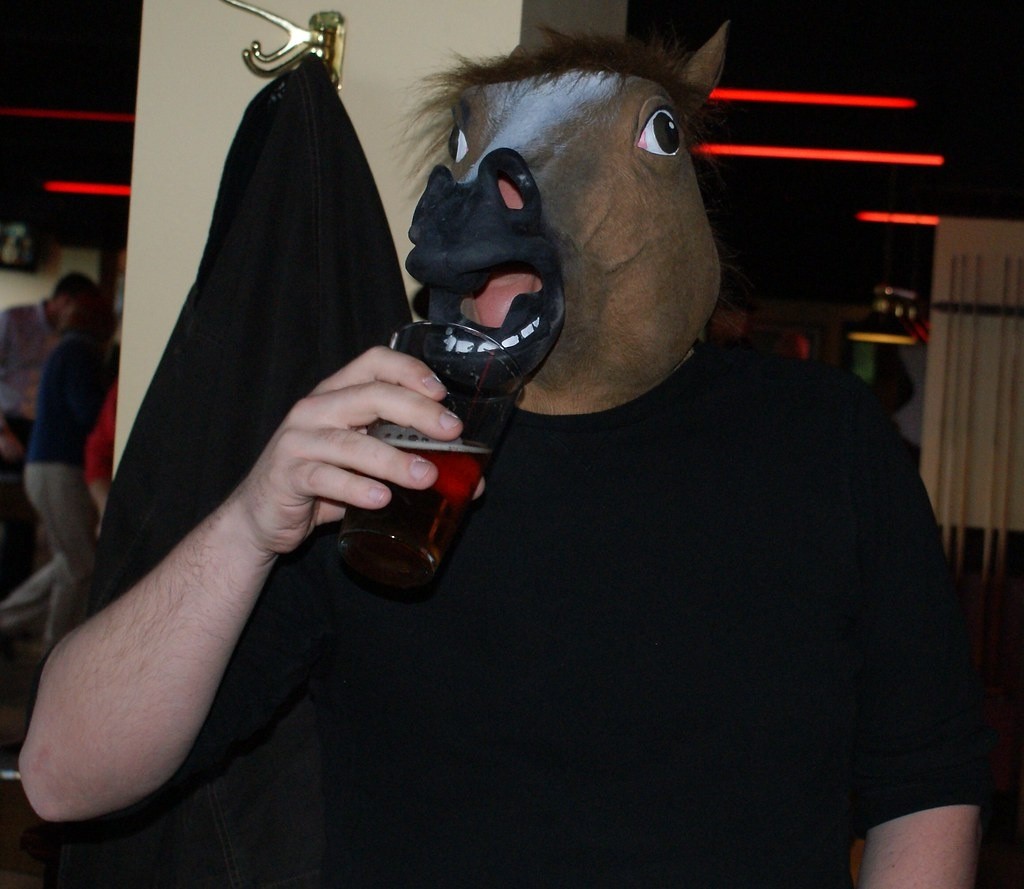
[845,309,915,343]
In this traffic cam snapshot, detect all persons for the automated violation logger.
[0,273,124,657]
[18,19,990,889]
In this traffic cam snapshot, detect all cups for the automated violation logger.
[335,322,523,588]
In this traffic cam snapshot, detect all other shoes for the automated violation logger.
[0,631,17,662]
